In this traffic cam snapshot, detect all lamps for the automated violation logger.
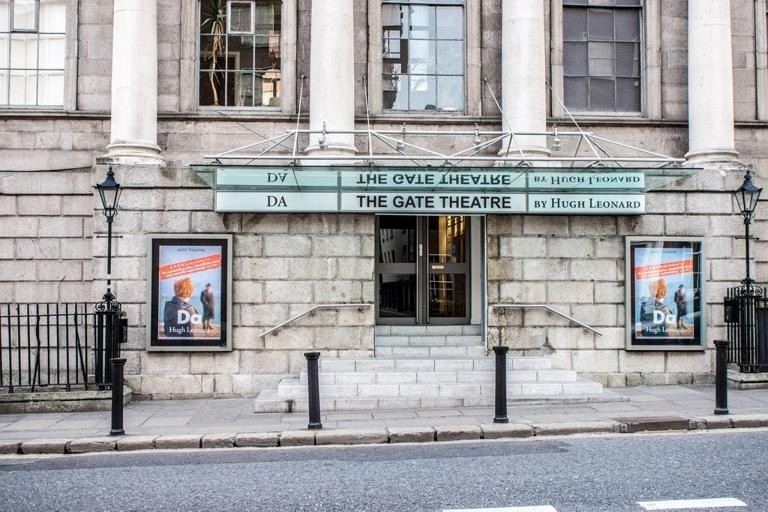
[552,122,561,151]
[472,123,483,154]
[318,121,329,151]
[396,122,407,151]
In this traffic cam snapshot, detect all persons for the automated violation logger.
[673,283,688,331]
[639,278,674,337]
[199,284,216,330]
[162,277,198,338]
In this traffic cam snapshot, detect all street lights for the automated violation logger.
[94,165,125,388]
[731,168,763,374]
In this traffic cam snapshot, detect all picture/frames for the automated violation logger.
[623,234,707,351]
[145,233,234,353]
